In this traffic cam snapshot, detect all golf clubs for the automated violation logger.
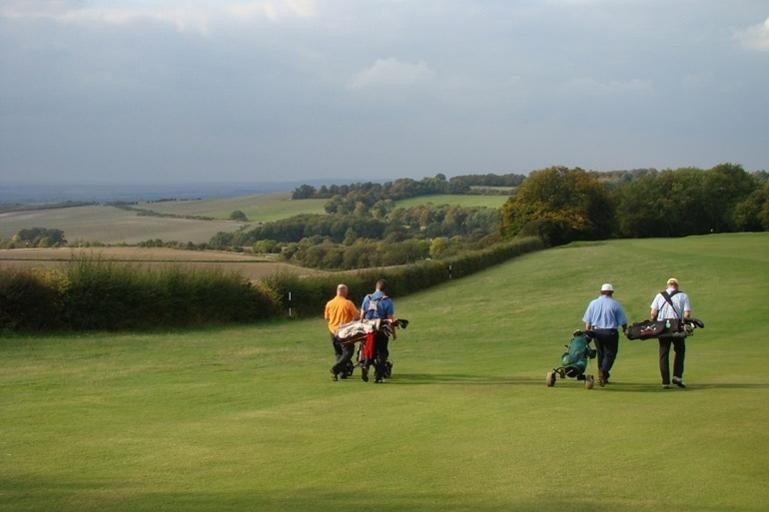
[680,322,696,335]
[381,319,409,337]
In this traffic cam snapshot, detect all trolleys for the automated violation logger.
[546,330,596,389]
[345,344,393,378]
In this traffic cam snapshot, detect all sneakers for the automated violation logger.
[661,376,686,389]
[598,371,608,387]
[329,367,384,383]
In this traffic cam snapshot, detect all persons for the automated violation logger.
[651,278,691,388]
[583,283,627,387]
[324,280,397,383]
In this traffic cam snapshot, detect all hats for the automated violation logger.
[601,283,614,291]
[666,278,679,287]
